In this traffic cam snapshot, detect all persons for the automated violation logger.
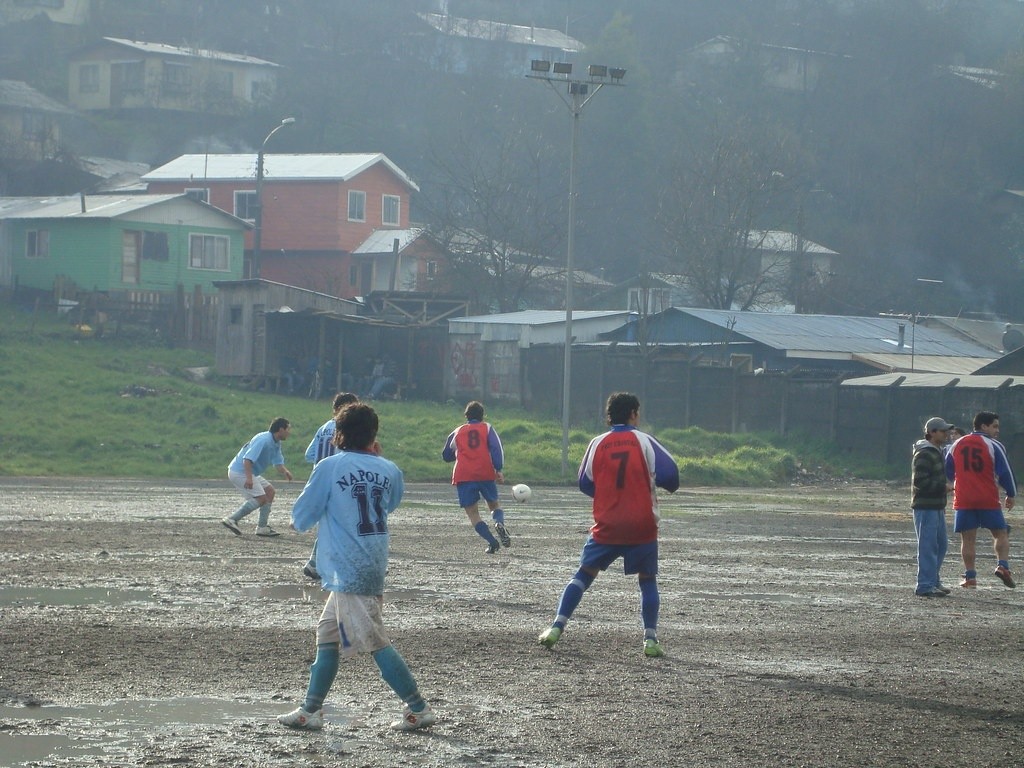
[276,402,438,731]
[538,391,680,658]
[302,392,390,580]
[442,400,511,555]
[941,426,1012,538]
[221,416,293,537]
[909,416,953,598]
[943,410,1017,589]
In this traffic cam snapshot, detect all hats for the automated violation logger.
[925,416,955,432]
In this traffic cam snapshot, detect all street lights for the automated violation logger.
[250,117,295,277]
[524,60,626,478]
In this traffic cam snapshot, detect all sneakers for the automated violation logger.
[959,578,976,588]
[302,561,322,579]
[495,522,512,547]
[994,566,1016,588]
[277,707,323,729]
[255,525,280,536]
[221,517,241,535]
[538,628,562,650]
[390,702,436,731]
[643,637,666,657]
[916,585,951,597]
[485,542,499,554]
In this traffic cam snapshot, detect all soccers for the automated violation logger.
[511,484,531,503]
